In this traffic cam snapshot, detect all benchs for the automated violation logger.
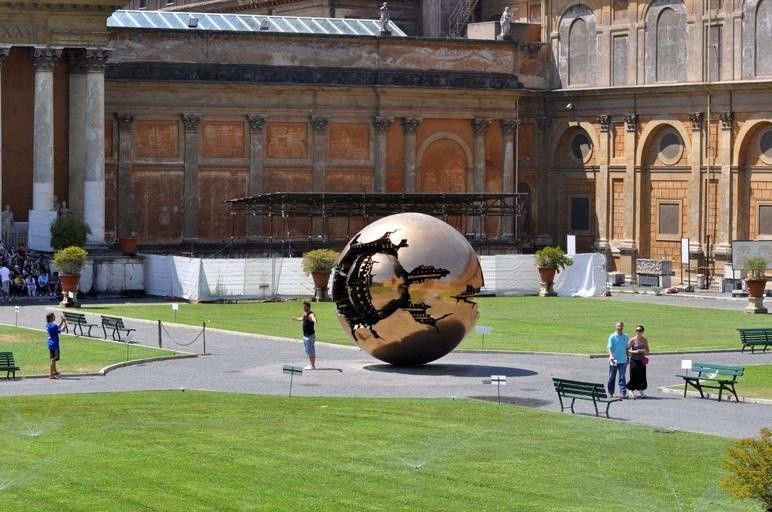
[1,352,20,381]
[736,329,771,354]
[553,378,622,418]
[675,362,745,402]
[61,312,136,343]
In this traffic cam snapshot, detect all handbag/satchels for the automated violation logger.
[641,357,649,365]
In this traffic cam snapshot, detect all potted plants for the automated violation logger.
[53,246,87,293]
[743,257,769,297]
[301,247,342,288]
[535,247,574,284]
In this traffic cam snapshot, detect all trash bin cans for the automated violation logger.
[697,274,705,289]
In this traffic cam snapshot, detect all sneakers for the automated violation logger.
[610,391,646,400]
[304,365,315,370]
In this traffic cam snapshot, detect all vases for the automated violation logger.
[119,235,139,254]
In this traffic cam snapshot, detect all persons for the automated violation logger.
[379,2,390,32]
[291,300,318,370]
[1,239,61,304]
[45,312,67,380]
[606,321,632,400]
[625,325,650,400]
[499,7,514,34]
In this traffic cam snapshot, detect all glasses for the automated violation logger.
[636,330,642,332]
